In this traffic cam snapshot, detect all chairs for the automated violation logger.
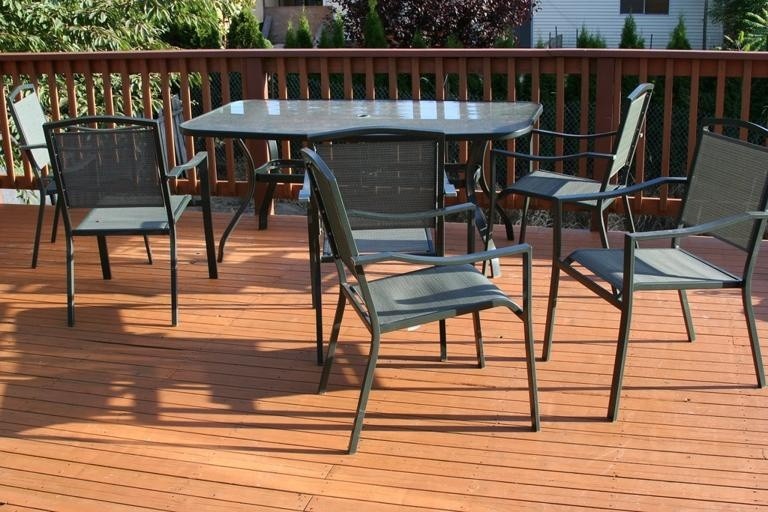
[541,116,768,423]
[300,124,457,367]
[7,83,153,269]
[42,115,217,325]
[300,147,540,453]
[480,82,654,301]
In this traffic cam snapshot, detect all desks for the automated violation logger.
[180,99,544,288]
